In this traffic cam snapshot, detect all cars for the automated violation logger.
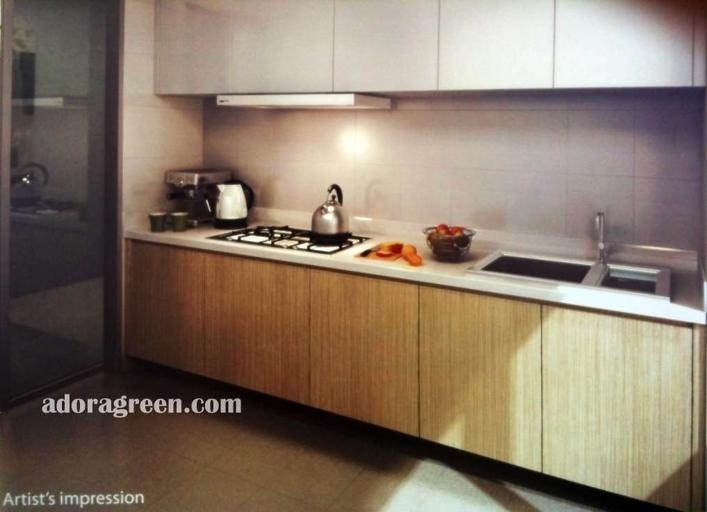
[169,212,188,231]
[149,211,167,232]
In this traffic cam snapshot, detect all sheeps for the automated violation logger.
[21,162,49,208]
[595,212,609,262]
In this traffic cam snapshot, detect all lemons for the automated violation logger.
[311,183,351,243]
[214,179,256,229]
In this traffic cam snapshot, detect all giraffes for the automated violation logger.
[204,226,372,256]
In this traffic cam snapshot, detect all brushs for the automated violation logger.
[165,169,233,228]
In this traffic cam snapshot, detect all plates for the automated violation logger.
[539,299,706,512]
[153,0,333,94]
[553,0,707,90]
[334,0,555,93]
[123,238,311,407]
[306,265,542,481]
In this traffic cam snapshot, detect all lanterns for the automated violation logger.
[401,245,416,256]
